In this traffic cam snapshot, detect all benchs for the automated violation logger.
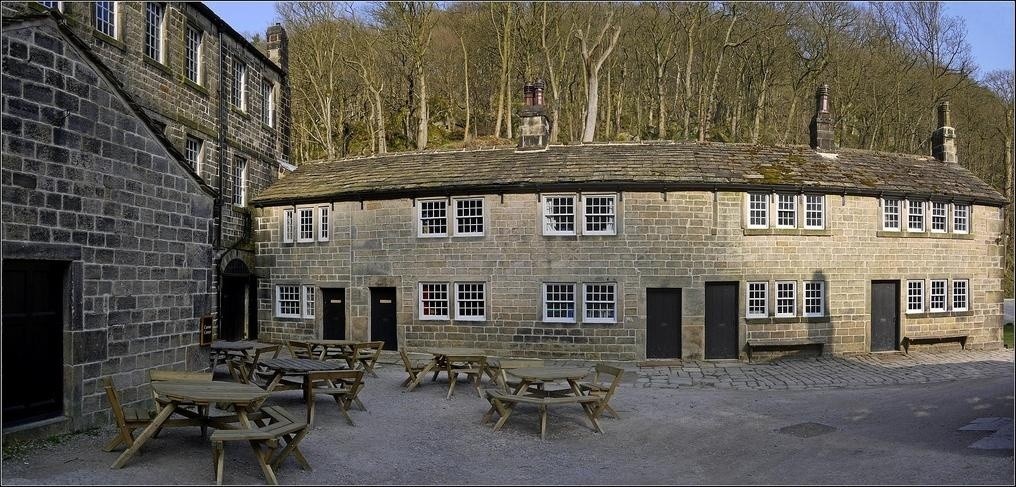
[210,405,313,485]
[211,340,501,413]
[481,388,605,440]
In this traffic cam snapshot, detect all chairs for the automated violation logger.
[580,363,624,420]
[149,368,213,437]
[100,375,154,457]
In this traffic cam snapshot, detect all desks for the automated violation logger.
[491,367,605,436]
[109,380,279,485]
